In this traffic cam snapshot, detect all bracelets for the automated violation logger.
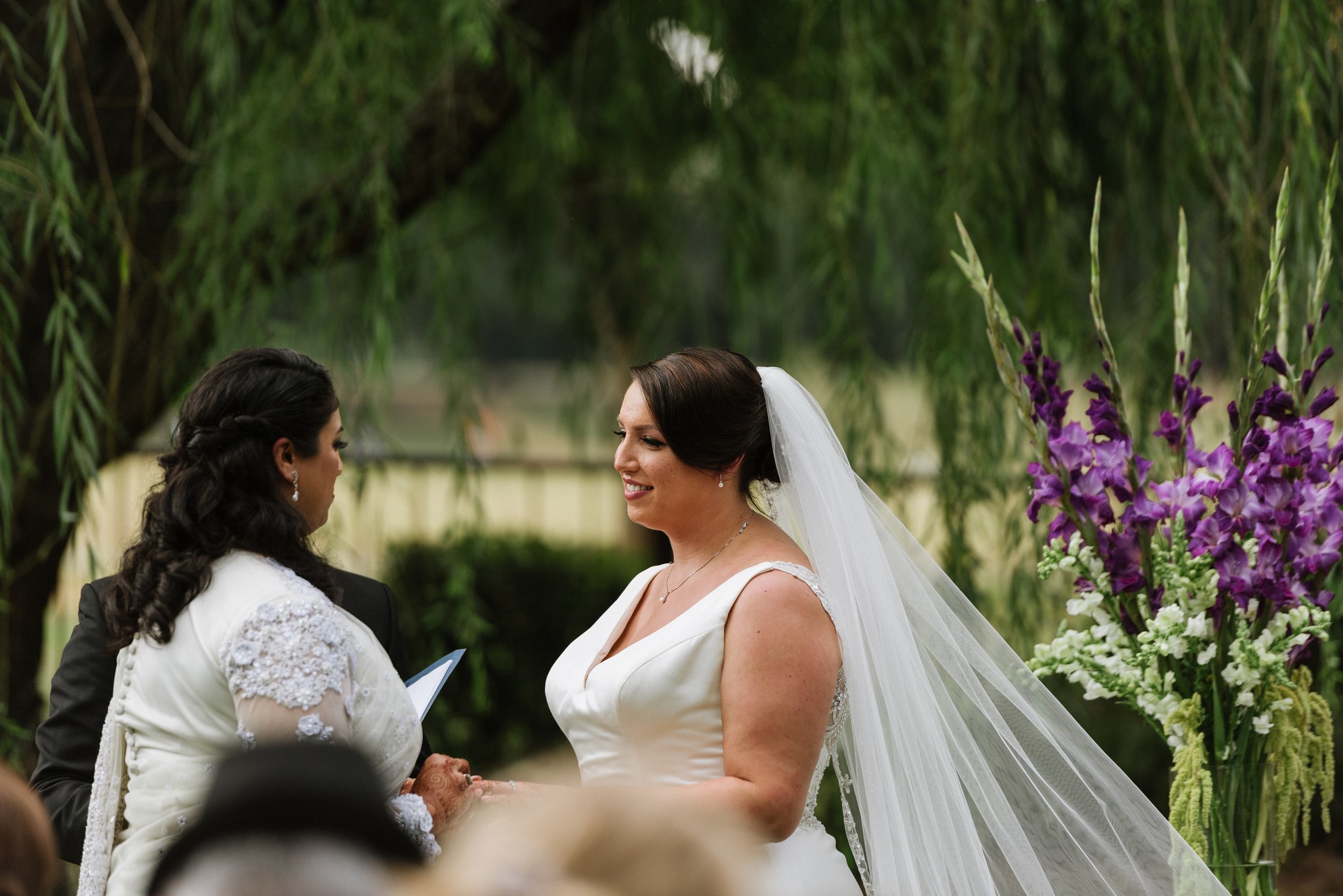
[509,780,518,799]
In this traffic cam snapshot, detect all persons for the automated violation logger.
[75,346,478,896]
[479,345,865,895]
[27,554,437,866]
[1,739,1342,896]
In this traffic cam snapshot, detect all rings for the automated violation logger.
[463,773,473,786]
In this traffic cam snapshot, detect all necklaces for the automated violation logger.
[659,510,756,603]
